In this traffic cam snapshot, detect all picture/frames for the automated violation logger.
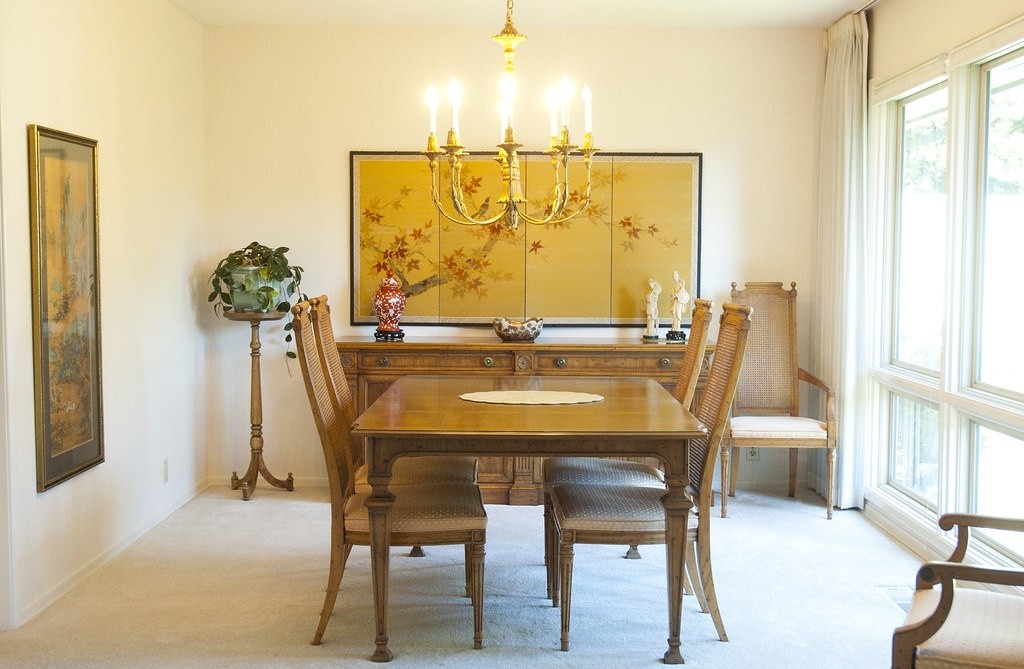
[27,125,106,492]
[349,149,703,331]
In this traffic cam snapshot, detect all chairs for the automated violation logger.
[290,302,488,651]
[550,305,756,650]
[891,519,1024,669]
[722,281,835,520]
[541,300,714,600]
[309,293,478,597]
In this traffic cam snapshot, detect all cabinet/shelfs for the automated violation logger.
[336,335,718,472]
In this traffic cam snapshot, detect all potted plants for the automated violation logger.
[206,241,311,372]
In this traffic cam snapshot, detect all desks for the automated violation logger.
[348,378,709,664]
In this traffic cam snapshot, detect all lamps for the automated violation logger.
[424,1,599,230]
